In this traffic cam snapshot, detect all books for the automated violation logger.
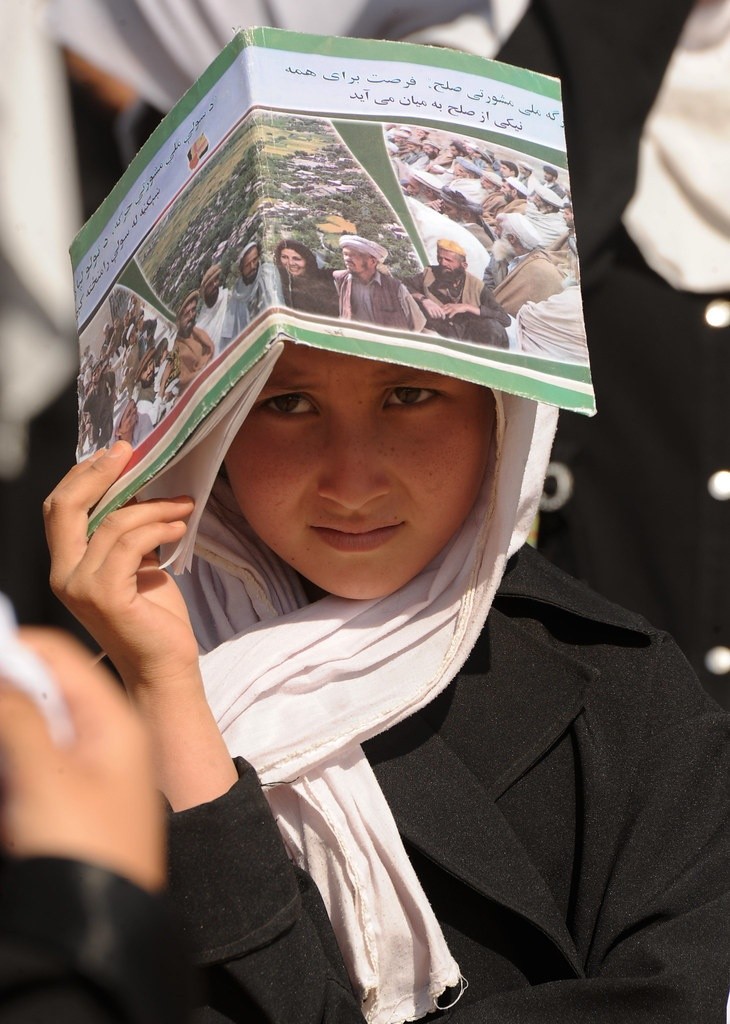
[66,26,598,584]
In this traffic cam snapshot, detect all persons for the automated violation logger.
[0,631,168,1024]
[42,341,730,1024]
[74,120,587,472]
[489,1,730,712]
